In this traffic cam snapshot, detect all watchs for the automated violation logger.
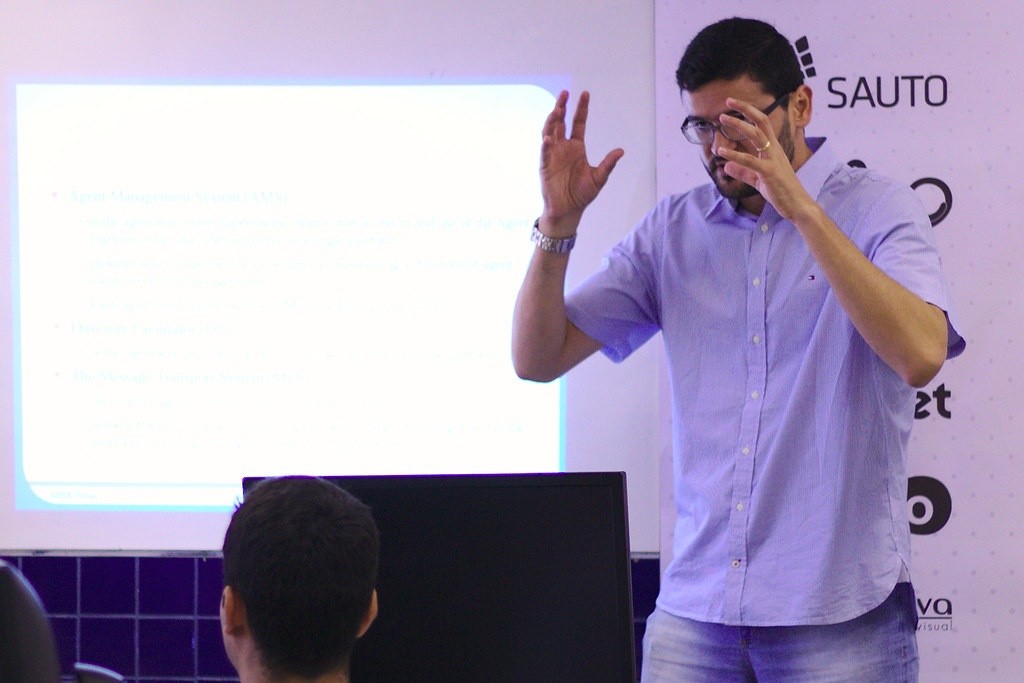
[530,217,577,254]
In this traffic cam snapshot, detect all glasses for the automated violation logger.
[681,95,787,145]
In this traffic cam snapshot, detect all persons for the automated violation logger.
[511,17,967,683]
[219,474,379,683]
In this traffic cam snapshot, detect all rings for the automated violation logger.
[756,140,771,152]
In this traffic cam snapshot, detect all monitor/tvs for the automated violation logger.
[242,470,636,683]
